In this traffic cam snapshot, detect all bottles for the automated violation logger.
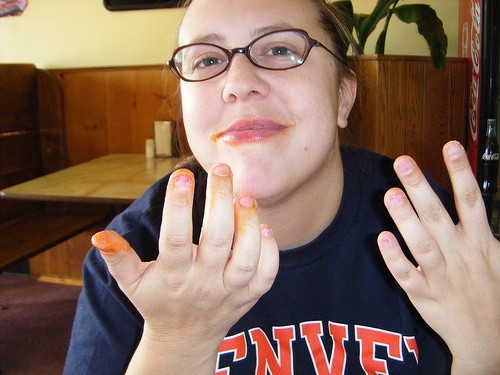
[478,118,500,224]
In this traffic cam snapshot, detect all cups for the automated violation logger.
[145,138,154,159]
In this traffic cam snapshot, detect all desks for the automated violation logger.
[0,151,188,206]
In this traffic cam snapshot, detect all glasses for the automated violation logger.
[167,28,349,82]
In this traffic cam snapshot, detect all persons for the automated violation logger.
[63,0,500,375]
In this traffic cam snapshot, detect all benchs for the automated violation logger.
[0,63,121,270]
